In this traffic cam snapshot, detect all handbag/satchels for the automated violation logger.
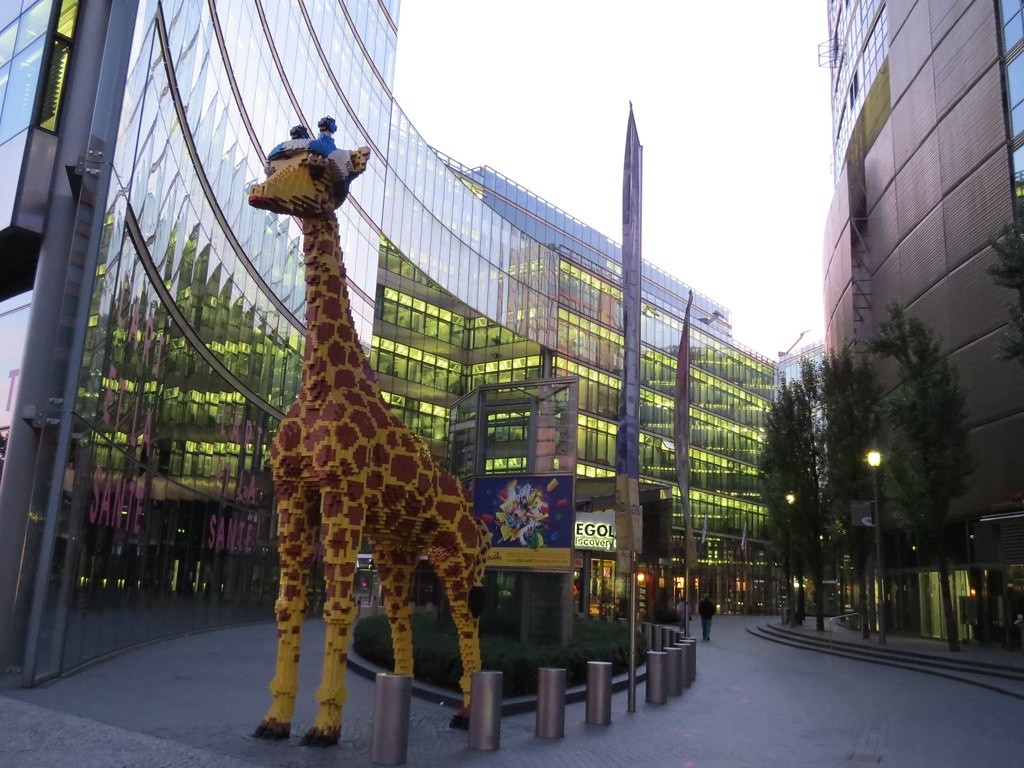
[689,614,692,621]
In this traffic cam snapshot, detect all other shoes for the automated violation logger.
[703,635,706,640]
[707,637,710,640]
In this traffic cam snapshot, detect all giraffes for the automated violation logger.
[249,117,491,748]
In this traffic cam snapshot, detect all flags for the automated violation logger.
[741,523,748,551]
[701,516,709,545]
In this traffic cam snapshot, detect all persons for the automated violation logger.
[698,593,715,640]
[676,598,693,637]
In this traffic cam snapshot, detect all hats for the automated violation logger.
[704,593,709,598]
